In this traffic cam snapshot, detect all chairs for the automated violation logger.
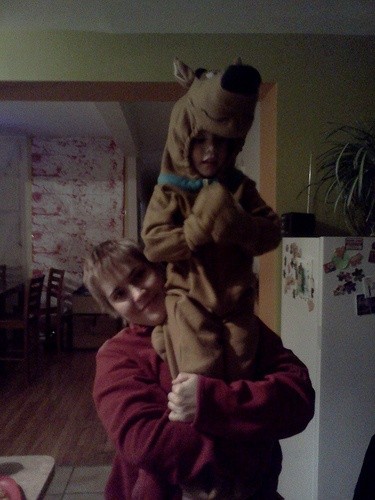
[0,264,65,387]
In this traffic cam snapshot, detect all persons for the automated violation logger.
[83,239,315,500]
[142,96,283,500]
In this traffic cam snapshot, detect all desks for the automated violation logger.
[0,277,27,354]
[0,455,57,500]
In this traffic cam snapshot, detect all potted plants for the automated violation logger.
[295,116,375,237]
